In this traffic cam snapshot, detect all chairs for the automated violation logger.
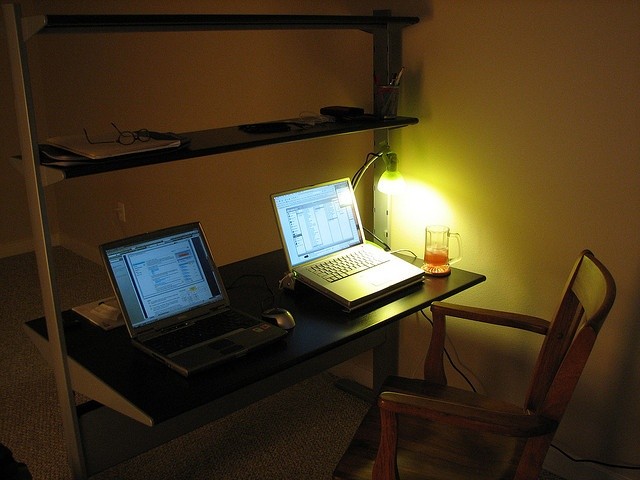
[331,250,616,480]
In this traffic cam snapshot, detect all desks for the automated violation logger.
[20,243,486,478]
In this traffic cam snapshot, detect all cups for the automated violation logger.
[378,85,399,119]
[424,224,463,274]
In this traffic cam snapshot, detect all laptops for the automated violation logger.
[97,221,289,380]
[271,176,426,310]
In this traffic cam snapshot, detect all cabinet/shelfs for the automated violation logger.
[3,3,419,189]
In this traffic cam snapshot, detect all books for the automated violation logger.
[41,129,182,161]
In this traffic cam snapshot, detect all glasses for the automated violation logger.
[83,122,150,145]
[223,274,275,315]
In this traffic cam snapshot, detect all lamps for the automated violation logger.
[349,139,404,197]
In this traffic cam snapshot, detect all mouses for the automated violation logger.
[260,307,296,330]
